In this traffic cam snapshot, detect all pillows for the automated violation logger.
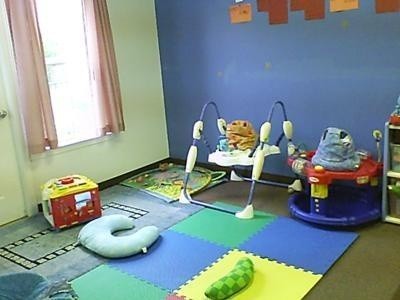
[77,214,161,260]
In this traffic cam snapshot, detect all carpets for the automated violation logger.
[0,184,206,291]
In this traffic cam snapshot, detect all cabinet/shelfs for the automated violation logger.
[380,116,400,227]
